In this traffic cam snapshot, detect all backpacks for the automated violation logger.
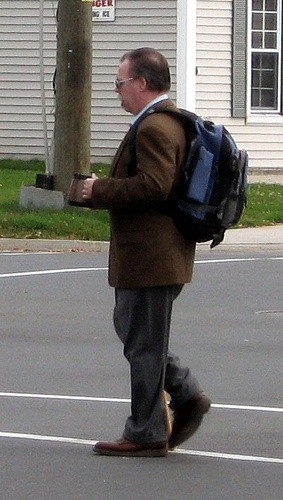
[128,108,250,249]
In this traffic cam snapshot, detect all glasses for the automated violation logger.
[114,76,139,89]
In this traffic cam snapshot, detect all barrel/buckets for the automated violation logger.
[35,174,54,191]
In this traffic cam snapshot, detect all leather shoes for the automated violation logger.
[167,390,211,450]
[92,433,168,457]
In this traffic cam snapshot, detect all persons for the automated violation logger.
[83,48,212,456]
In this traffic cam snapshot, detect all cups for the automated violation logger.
[66,173,97,208]
